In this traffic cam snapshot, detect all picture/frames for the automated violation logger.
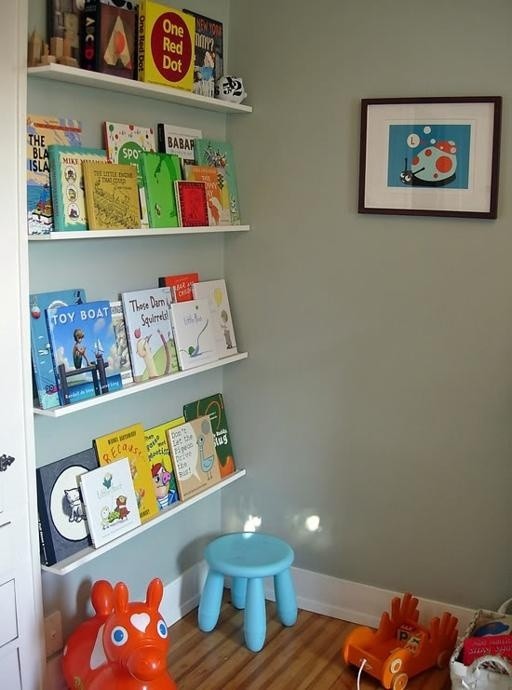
[357,95,503,221]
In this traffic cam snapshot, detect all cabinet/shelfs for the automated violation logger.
[24,61,247,578]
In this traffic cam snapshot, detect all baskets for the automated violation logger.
[449,597,511,688]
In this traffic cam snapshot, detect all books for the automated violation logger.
[49,0,226,105]
[29,271,244,412]
[27,116,244,237]
